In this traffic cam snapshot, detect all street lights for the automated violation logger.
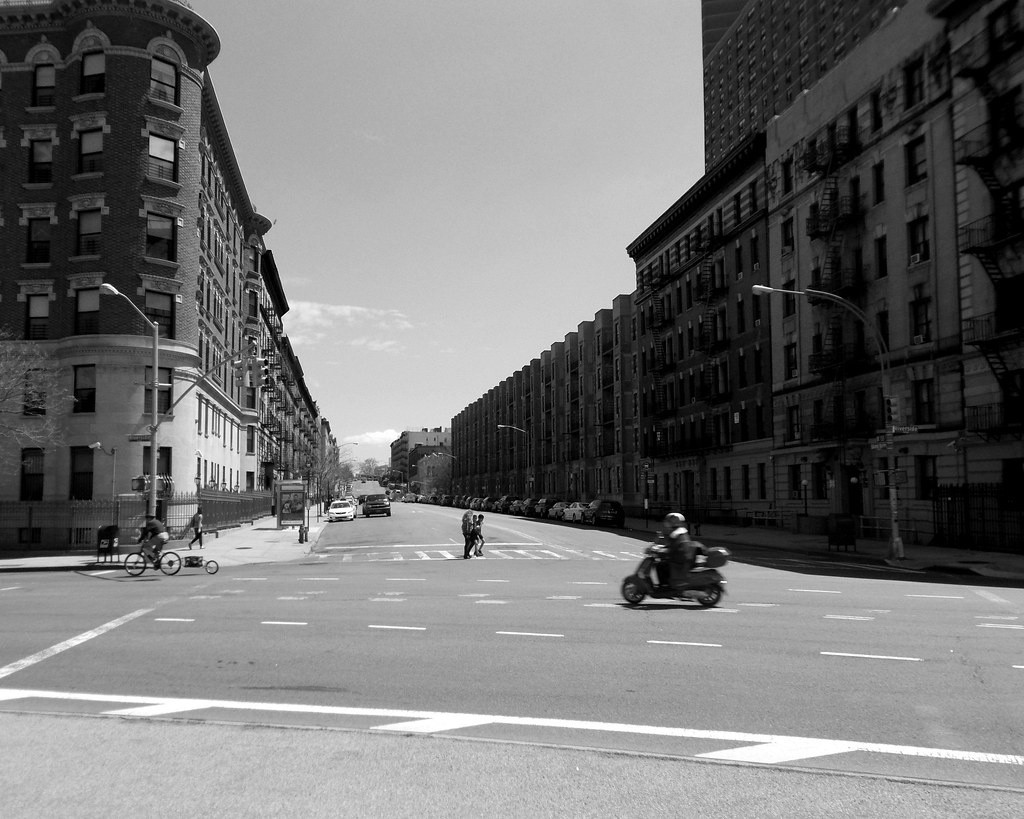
[99,284,159,525]
[801,480,809,518]
[750,284,904,560]
[497,425,530,498]
[392,470,403,495]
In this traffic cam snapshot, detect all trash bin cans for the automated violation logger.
[826,512,856,551]
[97,524,120,562]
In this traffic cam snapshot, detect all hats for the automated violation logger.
[145,512,157,516]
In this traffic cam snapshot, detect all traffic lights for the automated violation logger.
[234,359,251,387]
[251,357,270,388]
[131,478,146,491]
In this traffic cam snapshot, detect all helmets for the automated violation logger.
[664,513,686,527]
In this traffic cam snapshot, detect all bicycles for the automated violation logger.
[125,540,182,576]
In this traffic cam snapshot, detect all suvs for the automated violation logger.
[582,499,625,530]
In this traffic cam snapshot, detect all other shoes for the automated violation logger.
[475,552,482,556]
[464,555,470,559]
[468,554,472,558]
[153,557,158,562]
[478,550,484,555]
[188,543,192,550]
[200,546,205,549]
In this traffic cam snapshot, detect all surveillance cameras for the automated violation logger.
[89,442,101,449]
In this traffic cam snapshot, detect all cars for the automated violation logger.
[377,477,538,517]
[339,483,367,515]
[533,499,557,518]
[547,502,571,521]
[364,494,391,518]
[561,502,589,524]
[328,501,354,523]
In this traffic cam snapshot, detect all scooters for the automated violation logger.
[621,531,731,608]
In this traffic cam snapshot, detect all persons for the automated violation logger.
[137,513,169,571]
[291,494,301,511]
[462,509,485,559]
[656,512,691,584]
[188,508,205,550]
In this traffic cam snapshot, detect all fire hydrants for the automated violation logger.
[297,525,307,543]
